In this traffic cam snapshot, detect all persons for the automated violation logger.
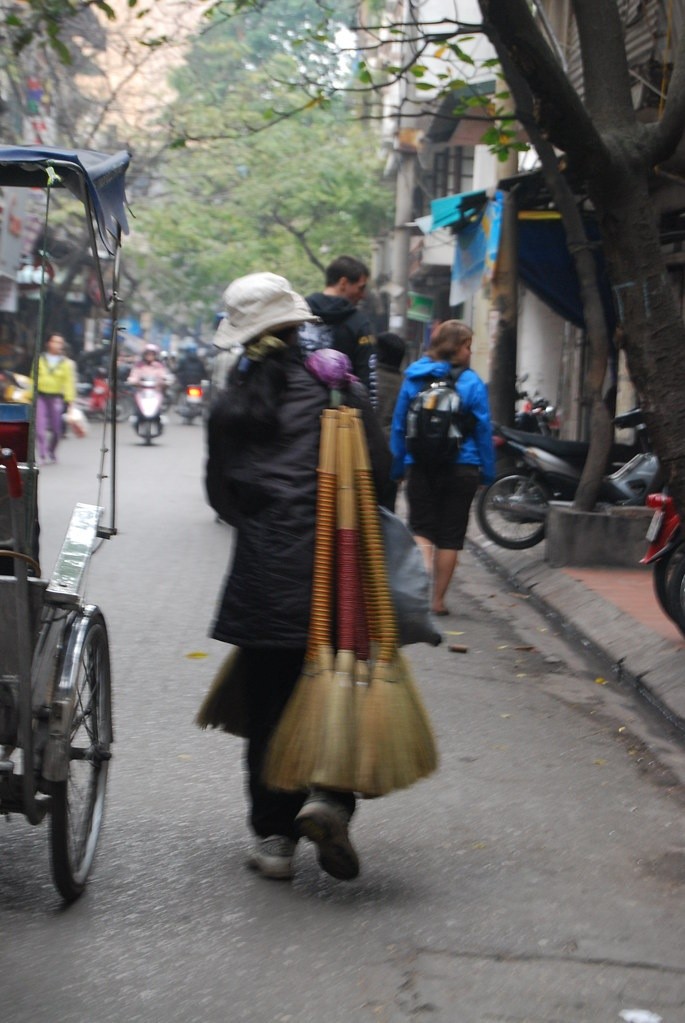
[303,254,497,615]
[204,274,390,880]
[34,330,209,463]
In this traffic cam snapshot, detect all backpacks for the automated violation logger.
[403,366,473,463]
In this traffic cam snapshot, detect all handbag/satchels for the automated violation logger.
[375,504,443,648]
[62,407,88,437]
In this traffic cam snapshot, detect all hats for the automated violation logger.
[211,270,320,350]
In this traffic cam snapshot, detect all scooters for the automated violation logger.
[124,380,169,447]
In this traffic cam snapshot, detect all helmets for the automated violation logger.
[142,344,157,355]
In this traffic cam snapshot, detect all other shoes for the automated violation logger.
[296,795,360,881]
[245,835,295,881]
[431,607,450,616]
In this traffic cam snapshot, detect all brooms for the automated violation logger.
[190,644,251,739]
[262,403,442,799]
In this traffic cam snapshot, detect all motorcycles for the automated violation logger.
[640,485,685,634]
[473,393,667,550]
[75,365,132,424]
[174,382,204,426]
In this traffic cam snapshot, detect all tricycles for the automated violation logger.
[0,141,137,913]
